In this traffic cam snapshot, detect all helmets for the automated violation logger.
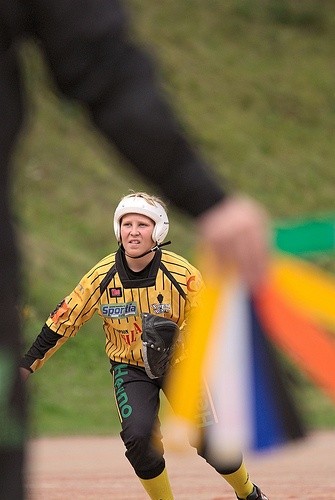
[113,194,169,243]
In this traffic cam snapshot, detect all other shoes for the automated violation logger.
[235,483,268,500]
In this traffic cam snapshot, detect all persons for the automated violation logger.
[19,191,268,500]
[0,0,271,500]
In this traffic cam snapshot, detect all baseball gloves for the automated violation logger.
[141,312,181,379]
